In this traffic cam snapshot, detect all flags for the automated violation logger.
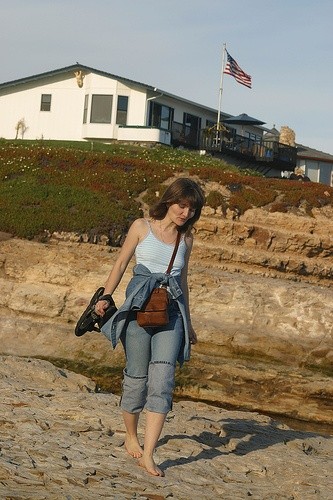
[223,48,252,89]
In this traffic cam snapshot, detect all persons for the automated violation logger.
[75,178,206,476]
[72,70,83,88]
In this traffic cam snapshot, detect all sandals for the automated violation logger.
[96,295,117,329]
[75,287,105,337]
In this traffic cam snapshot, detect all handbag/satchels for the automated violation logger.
[137,286,169,327]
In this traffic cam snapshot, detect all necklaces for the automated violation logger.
[160,220,177,244]
